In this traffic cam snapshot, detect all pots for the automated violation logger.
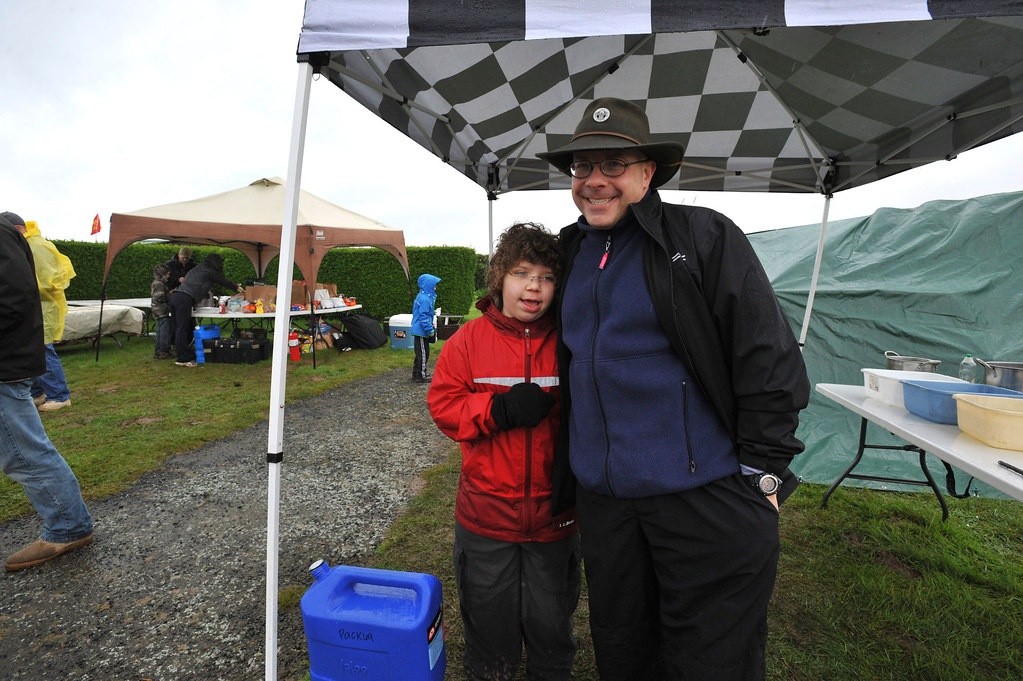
[885,350,942,374]
[976,357,1023,392]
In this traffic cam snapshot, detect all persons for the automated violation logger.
[0,211,71,410]
[151,263,176,360]
[169,253,247,367]
[429,224,582,681]
[266,0,1023,681]
[412,274,441,383]
[164,247,197,352]
[534,97,811,681]
[0,219,94,570]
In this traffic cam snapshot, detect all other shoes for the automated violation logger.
[412,373,433,383]
[37,399,71,412]
[174,360,198,367]
[161,353,175,359]
[33,393,48,404]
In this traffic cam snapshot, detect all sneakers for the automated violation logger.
[5,532,94,570]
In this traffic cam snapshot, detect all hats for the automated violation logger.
[178,247,192,256]
[0,211,25,225]
[536,96,685,186]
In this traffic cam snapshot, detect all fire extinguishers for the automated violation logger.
[289,328,300,361]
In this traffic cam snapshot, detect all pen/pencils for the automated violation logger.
[998,460,1023,478]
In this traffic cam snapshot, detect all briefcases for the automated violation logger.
[194,324,269,365]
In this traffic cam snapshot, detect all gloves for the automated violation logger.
[489,383,556,432]
[429,334,436,342]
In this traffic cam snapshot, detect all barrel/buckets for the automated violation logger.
[194,325,221,364]
[301,558,447,681]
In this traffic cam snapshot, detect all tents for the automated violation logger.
[96,177,414,362]
[745,191,1023,505]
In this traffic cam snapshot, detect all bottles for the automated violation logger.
[959,353,976,384]
[316,334,322,351]
[219,299,226,314]
[300,333,312,359]
[256,299,263,314]
[298,328,303,343]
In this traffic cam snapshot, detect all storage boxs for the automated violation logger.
[246,283,338,304]
[388,313,438,348]
[860,368,1023,450]
[202,328,270,364]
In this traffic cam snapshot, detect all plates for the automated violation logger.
[197,306,219,314]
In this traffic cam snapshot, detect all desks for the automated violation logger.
[814,383,1023,523]
[55,297,153,348]
[191,305,363,350]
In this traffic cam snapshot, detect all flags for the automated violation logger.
[91,214,100,235]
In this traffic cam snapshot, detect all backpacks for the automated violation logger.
[341,312,388,349]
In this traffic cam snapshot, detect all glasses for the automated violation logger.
[507,269,559,288]
[567,155,653,179]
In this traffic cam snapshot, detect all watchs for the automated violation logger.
[745,472,782,496]
